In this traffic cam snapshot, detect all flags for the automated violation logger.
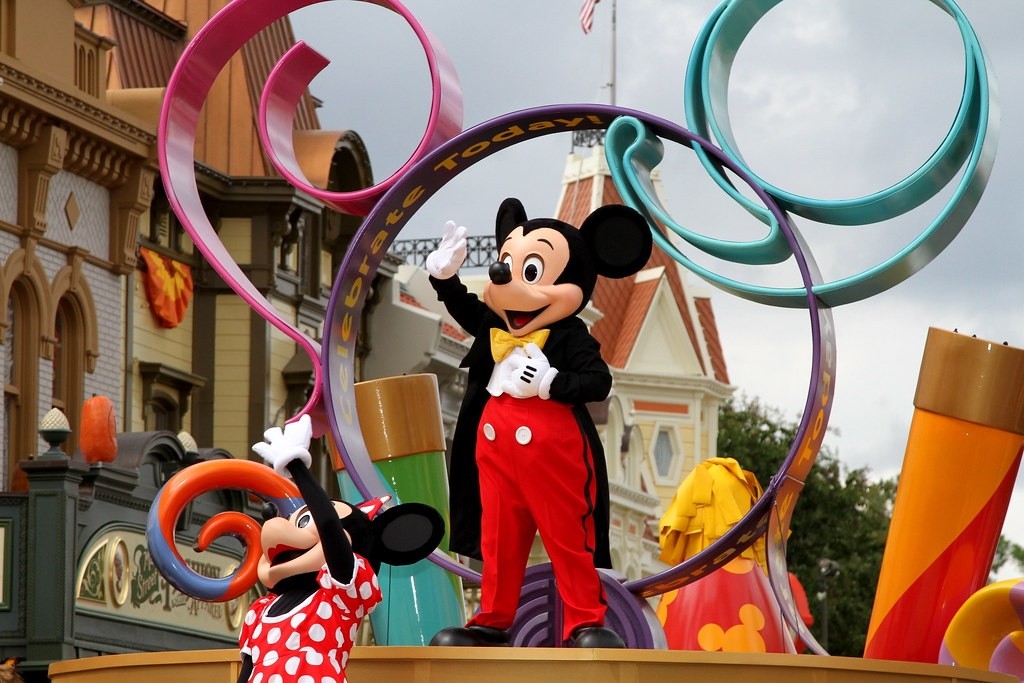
[578,0,600,34]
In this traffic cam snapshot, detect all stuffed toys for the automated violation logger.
[240,416,446,683]
[426,196,654,648]
